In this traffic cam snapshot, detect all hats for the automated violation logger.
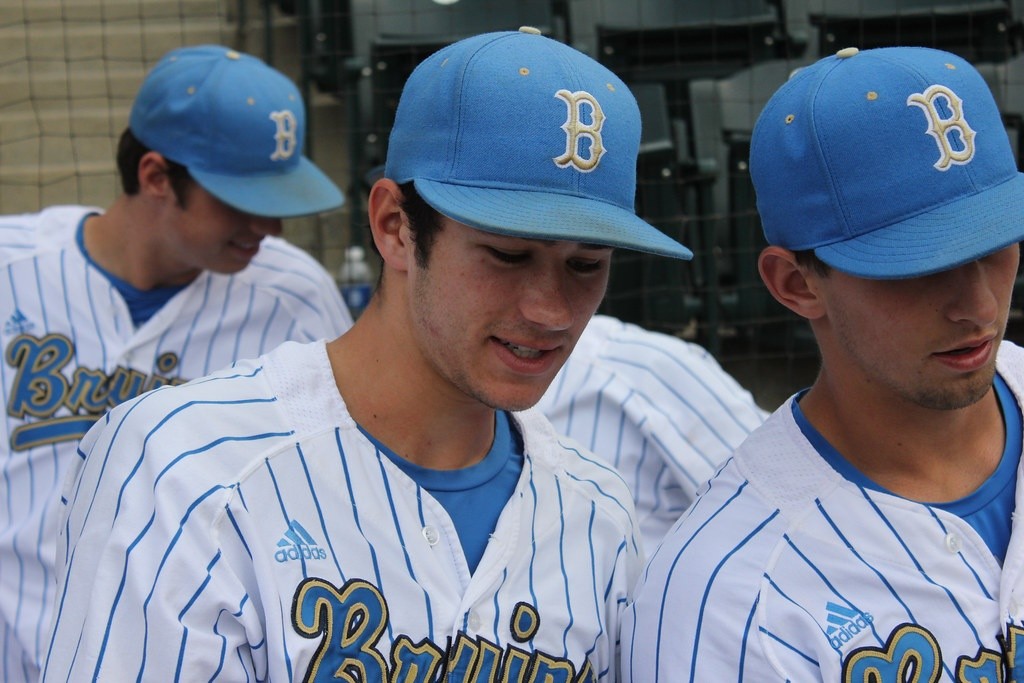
[128,44,344,217]
[748,46,1024,281]
[383,26,694,260]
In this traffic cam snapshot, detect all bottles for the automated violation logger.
[338,246,372,321]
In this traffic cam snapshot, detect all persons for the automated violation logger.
[1,46,353,683]
[620,43,1024,683]
[42,27,646,682]
